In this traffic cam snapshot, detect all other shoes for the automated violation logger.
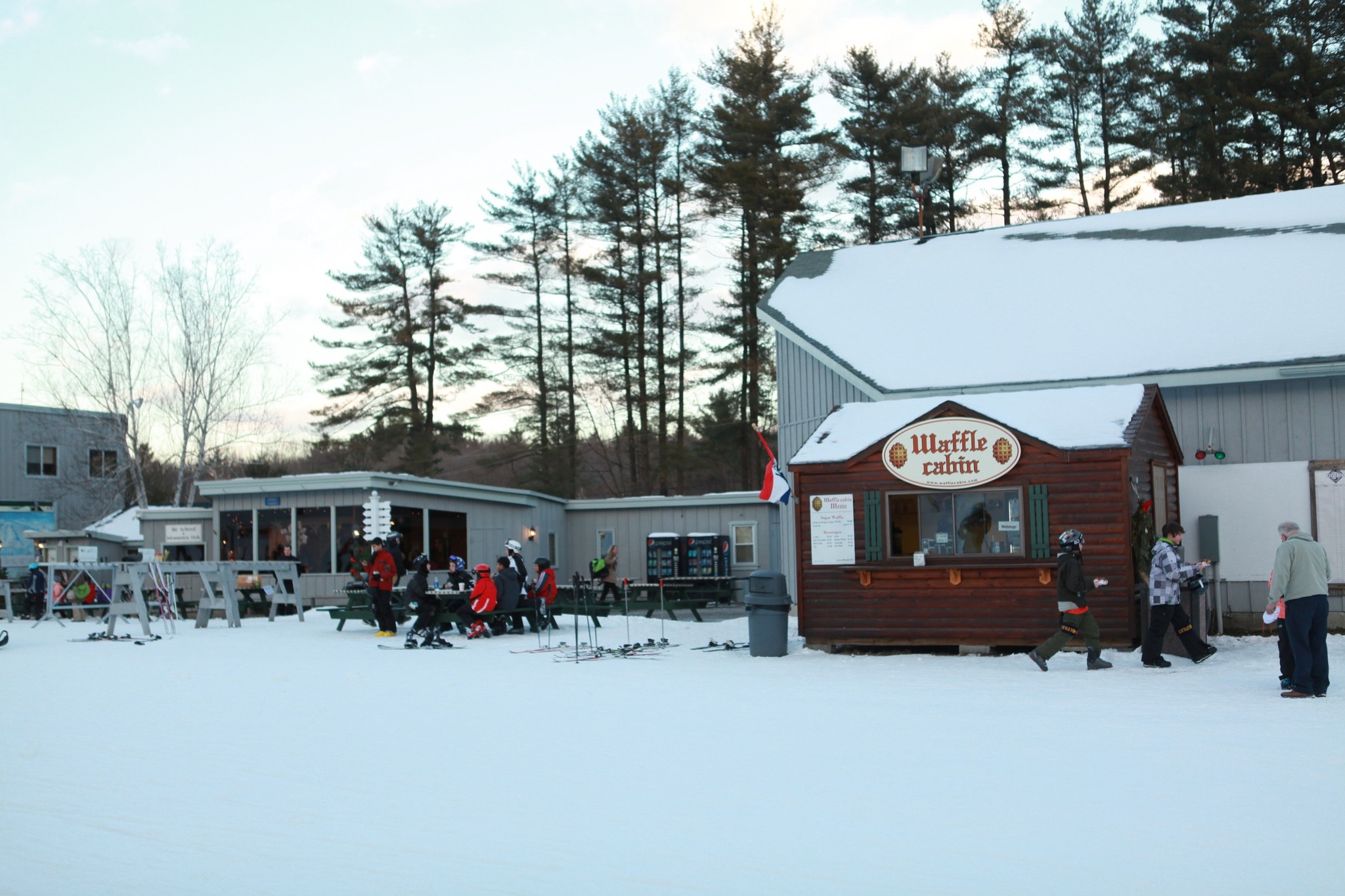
[404,617,549,649]
[375,630,385,637]
[1313,692,1327,697]
[383,630,396,637]
[398,616,412,625]
[363,619,377,627]
[1281,688,1315,698]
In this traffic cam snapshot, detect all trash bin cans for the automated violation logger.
[744,570,794,657]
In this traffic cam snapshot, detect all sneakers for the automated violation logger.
[1028,649,1048,672]
[1280,678,1293,690]
[1143,658,1171,668]
[1087,657,1113,669]
[1195,646,1217,663]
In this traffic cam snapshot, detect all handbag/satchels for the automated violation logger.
[73,582,91,599]
[66,590,77,600]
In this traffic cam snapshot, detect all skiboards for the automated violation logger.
[508,645,596,653]
[552,642,682,662]
[378,644,468,650]
[66,633,152,643]
[689,641,749,653]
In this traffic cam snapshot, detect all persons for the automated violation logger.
[939,498,958,545]
[403,554,453,648]
[489,556,523,635]
[59,559,94,617]
[504,539,529,634]
[434,556,474,634]
[1029,529,1112,670]
[363,532,411,627]
[528,558,557,630]
[361,537,397,637]
[272,544,303,617]
[600,545,620,601]
[1265,521,1333,698]
[1140,523,1217,669]
[463,564,497,638]
[19,563,45,621]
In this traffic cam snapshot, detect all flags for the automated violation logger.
[756,429,791,505]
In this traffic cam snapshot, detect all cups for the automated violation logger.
[458,583,465,591]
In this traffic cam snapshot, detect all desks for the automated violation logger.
[629,584,694,621]
[663,576,737,607]
[557,585,603,616]
[571,577,642,615]
[425,590,490,638]
[333,587,409,631]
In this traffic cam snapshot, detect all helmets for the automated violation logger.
[504,540,521,553]
[1188,575,1208,595]
[533,557,550,572]
[1058,529,1084,552]
[412,556,430,575]
[28,563,39,571]
[386,532,400,546]
[449,557,464,571]
[473,564,490,577]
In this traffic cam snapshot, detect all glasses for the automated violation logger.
[505,538,511,545]
[450,556,457,561]
[472,569,476,575]
[422,553,427,558]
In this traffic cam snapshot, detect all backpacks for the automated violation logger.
[589,555,614,580]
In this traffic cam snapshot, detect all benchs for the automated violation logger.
[316,588,742,638]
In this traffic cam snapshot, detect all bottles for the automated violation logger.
[434,577,439,592]
